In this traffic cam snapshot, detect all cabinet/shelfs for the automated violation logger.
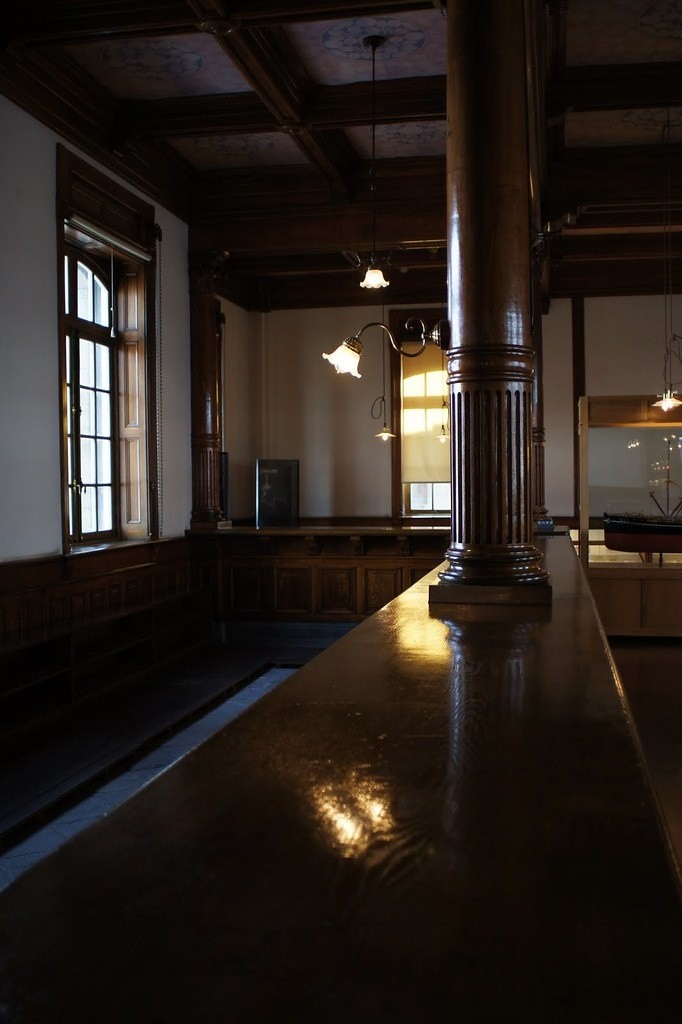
[572,396,682,637]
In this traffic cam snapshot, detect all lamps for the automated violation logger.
[371,304,396,440]
[360,34,389,288]
[651,125,679,411]
[322,316,450,377]
[655,84,682,408]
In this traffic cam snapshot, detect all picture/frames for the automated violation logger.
[256,458,300,530]
